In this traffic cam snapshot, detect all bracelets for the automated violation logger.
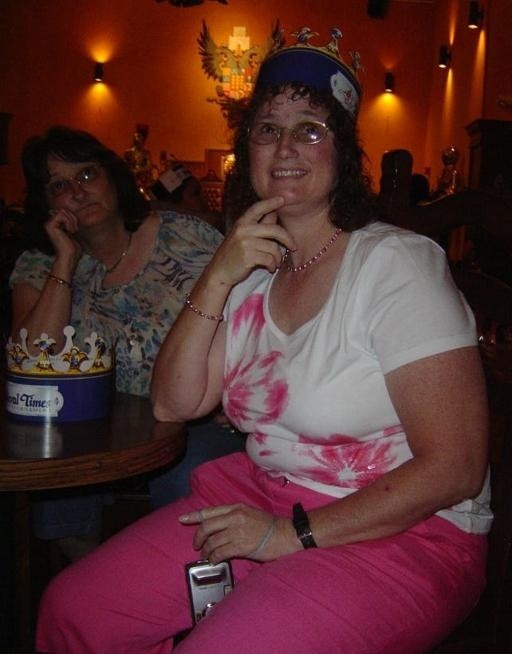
[42,271,71,292]
[289,503,319,556]
[183,298,226,324]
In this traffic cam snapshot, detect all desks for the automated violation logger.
[1,381,191,537]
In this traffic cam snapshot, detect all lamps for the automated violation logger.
[85,52,109,87]
[465,6,484,31]
[381,70,402,96]
[434,40,454,71]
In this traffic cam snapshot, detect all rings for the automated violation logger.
[49,208,58,215]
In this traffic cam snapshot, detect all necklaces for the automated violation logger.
[92,229,131,280]
[278,227,343,272]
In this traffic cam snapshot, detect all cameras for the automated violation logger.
[185,560,234,626]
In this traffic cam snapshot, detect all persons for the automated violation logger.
[33,83,494,653]
[11,127,247,565]
[146,117,511,373]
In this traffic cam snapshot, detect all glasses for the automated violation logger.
[246,119,336,144]
[47,165,105,197]
